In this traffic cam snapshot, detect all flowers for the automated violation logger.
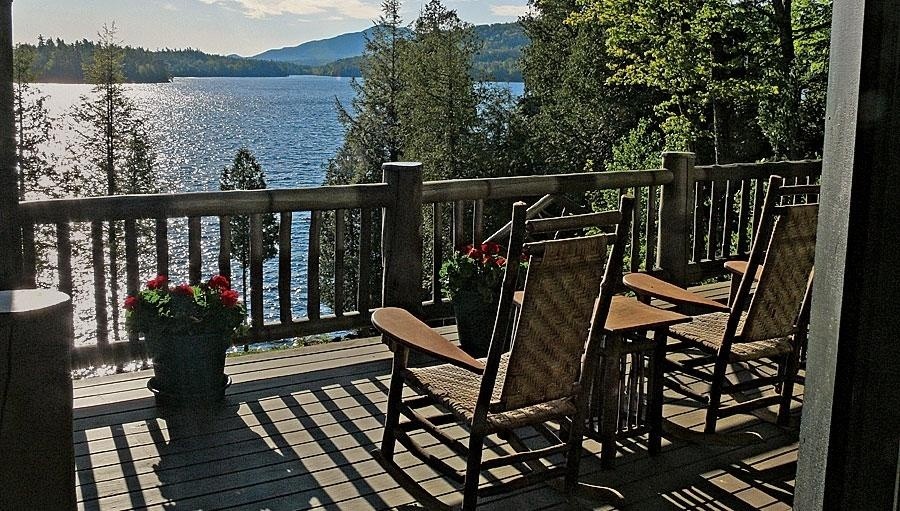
[124,274,247,334]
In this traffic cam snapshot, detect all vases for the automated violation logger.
[148,337,230,410]
